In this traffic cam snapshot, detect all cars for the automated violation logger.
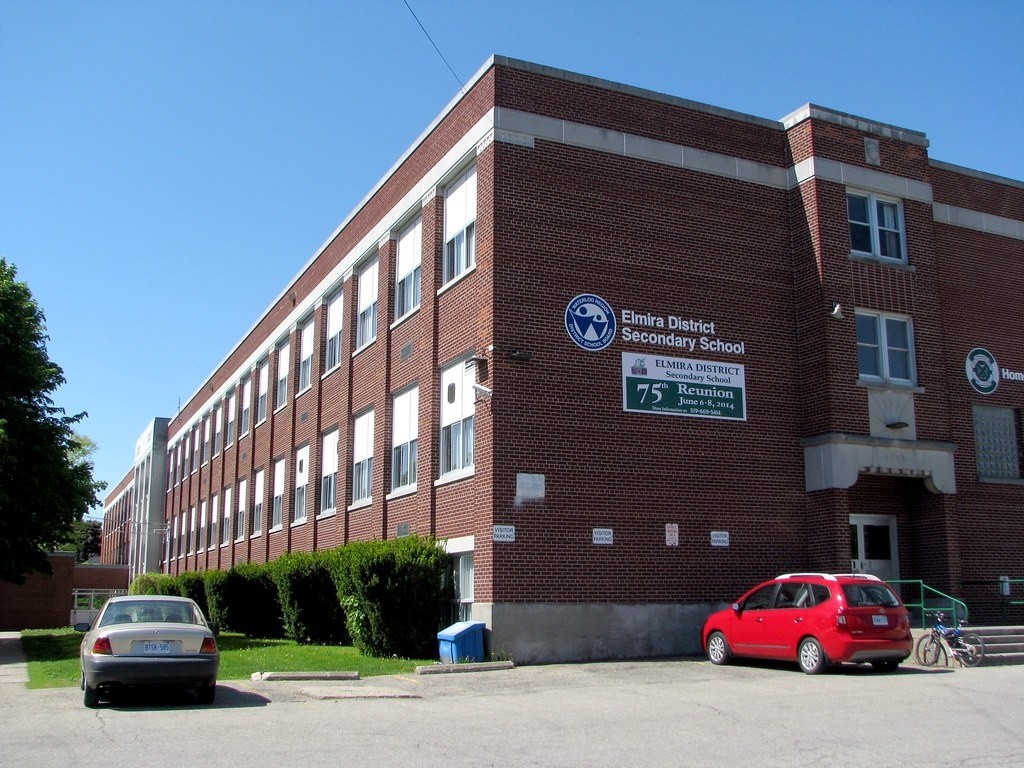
[74,595,220,708]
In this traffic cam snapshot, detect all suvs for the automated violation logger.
[701,573,914,675]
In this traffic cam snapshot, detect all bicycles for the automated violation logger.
[915,611,985,668]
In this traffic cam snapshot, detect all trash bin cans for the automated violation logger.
[436,620,487,664]
